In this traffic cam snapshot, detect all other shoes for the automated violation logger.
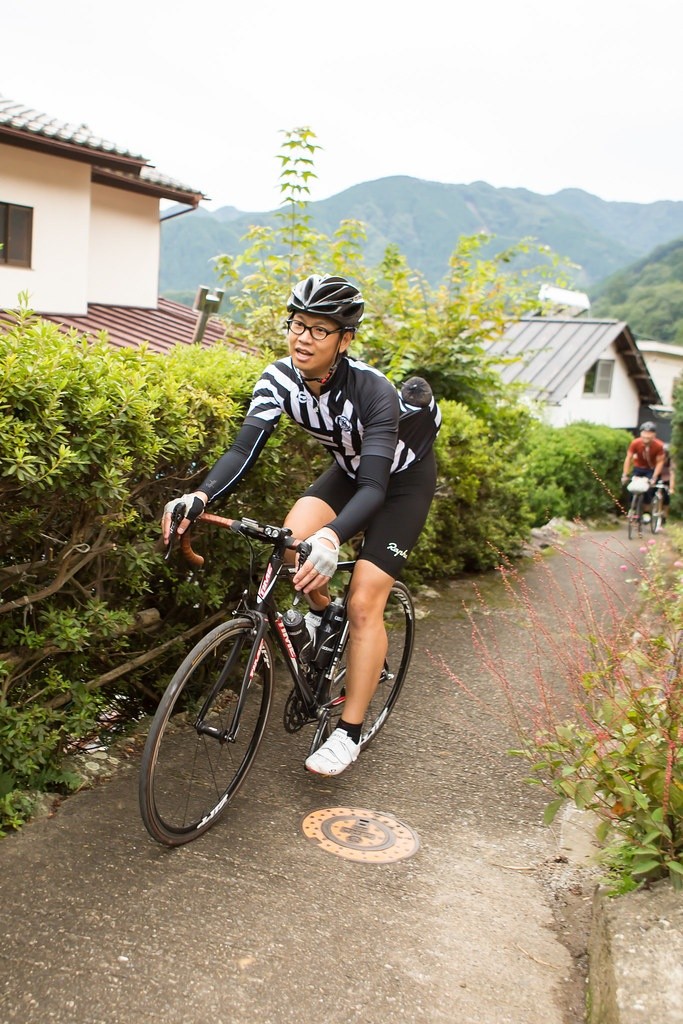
[642,513,651,523]
[628,509,636,521]
[662,517,666,523]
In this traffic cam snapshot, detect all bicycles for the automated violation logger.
[622,476,669,540]
[138,501,416,848]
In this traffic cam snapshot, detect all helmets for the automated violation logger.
[639,421,657,432]
[665,444,669,451]
[287,272,365,334]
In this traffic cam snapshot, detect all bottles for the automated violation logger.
[284,607,313,664]
[313,596,346,670]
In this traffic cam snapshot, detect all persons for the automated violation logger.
[162,275,436,776]
[621,421,675,524]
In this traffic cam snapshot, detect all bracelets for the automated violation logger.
[622,475,629,478]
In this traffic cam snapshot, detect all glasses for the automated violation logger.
[286,313,355,340]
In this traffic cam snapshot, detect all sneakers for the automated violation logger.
[304,728,362,778]
[303,594,343,642]
